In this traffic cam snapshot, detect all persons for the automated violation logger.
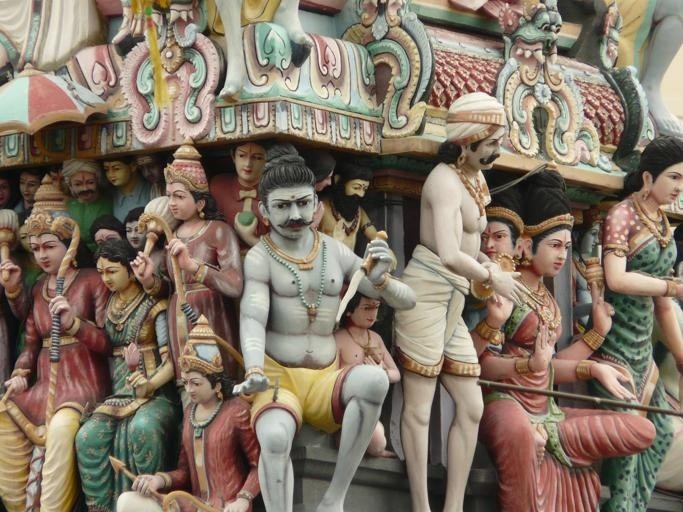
[0,94,682,510]
